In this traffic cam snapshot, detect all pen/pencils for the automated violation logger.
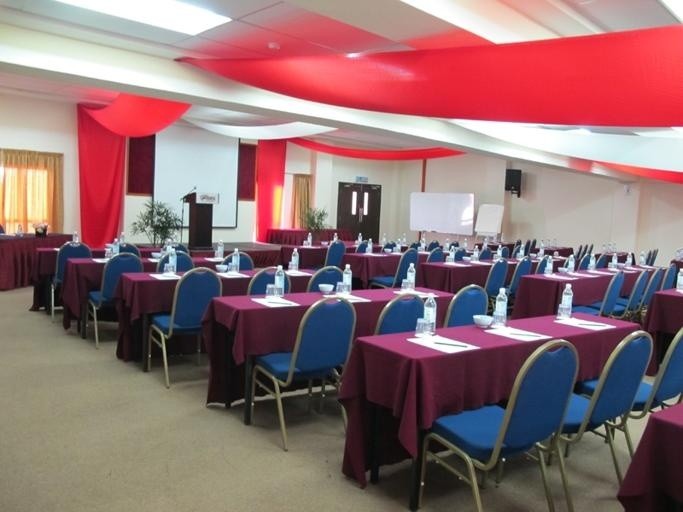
[579,323,604,327]
[268,301,293,305]
[435,342,468,347]
[511,333,542,337]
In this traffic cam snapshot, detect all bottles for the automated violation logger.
[275,265,285,297]
[343,265,352,295]
[492,287,506,326]
[561,283,573,314]
[69,232,658,275]
[421,294,437,336]
[407,263,416,291]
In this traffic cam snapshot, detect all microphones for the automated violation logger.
[189,186,196,194]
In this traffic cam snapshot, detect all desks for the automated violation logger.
[198,287,456,410]
[336,310,644,508]
[616,401,681,510]
[268,228,353,245]
[0,233,73,291]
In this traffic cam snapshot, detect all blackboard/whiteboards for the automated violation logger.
[409,191,475,236]
[474,203,504,234]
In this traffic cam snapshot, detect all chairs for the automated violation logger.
[416,340,579,512]
[551,328,655,511]
[563,326,681,462]
[33,241,681,388]
[248,296,358,451]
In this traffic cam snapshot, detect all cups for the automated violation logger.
[336,282,345,294]
[402,279,410,291]
[266,285,275,298]
[414,319,430,338]
[556,304,571,320]
[493,312,505,327]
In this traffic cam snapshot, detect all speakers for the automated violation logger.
[506,169,521,191]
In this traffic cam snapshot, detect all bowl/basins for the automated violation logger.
[318,284,334,293]
[472,314,493,328]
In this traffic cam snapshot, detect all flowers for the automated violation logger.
[30,222,49,234]
